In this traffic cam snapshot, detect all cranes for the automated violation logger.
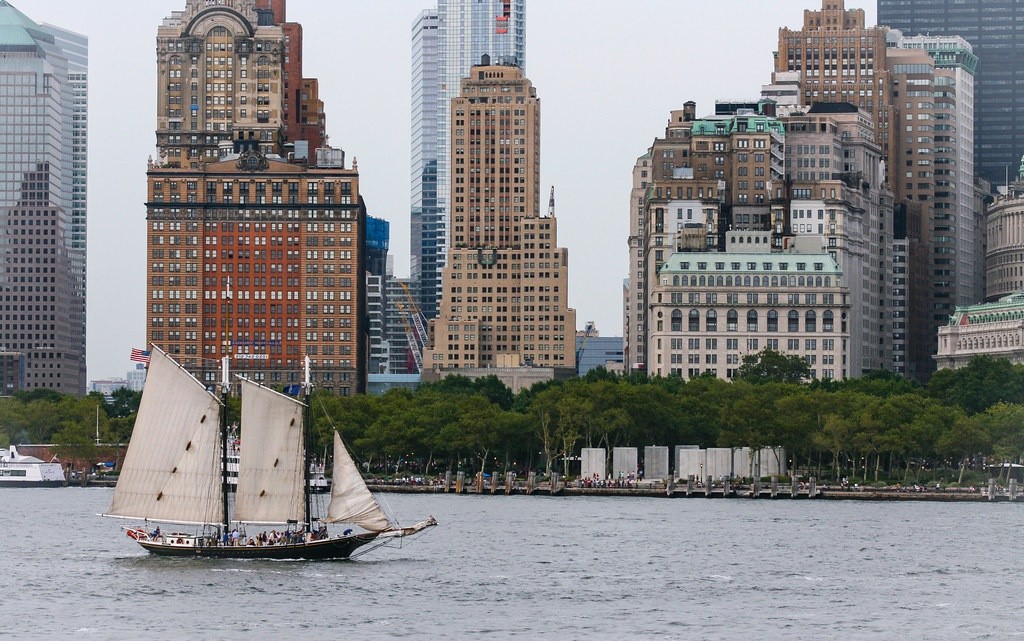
[394,281,428,375]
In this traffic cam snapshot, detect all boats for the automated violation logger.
[218,421,329,495]
[0,444,67,488]
[88,479,118,488]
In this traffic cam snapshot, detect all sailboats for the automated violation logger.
[92,343,438,565]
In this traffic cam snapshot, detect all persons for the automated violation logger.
[147,526,161,541]
[563,479,568,486]
[548,470,553,484]
[692,474,695,482]
[840,475,851,492]
[499,473,504,485]
[468,475,478,487]
[2,459,7,466]
[696,473,699,481]
[579,470,652,489]
[798,481,805,493]
[208,518,337,548]
[968,476,1006,494]
[372,467,446,490]
[488,476,492,486]
[894,480,943,495]
[733,481,739,493]
[71,471,76,479]
